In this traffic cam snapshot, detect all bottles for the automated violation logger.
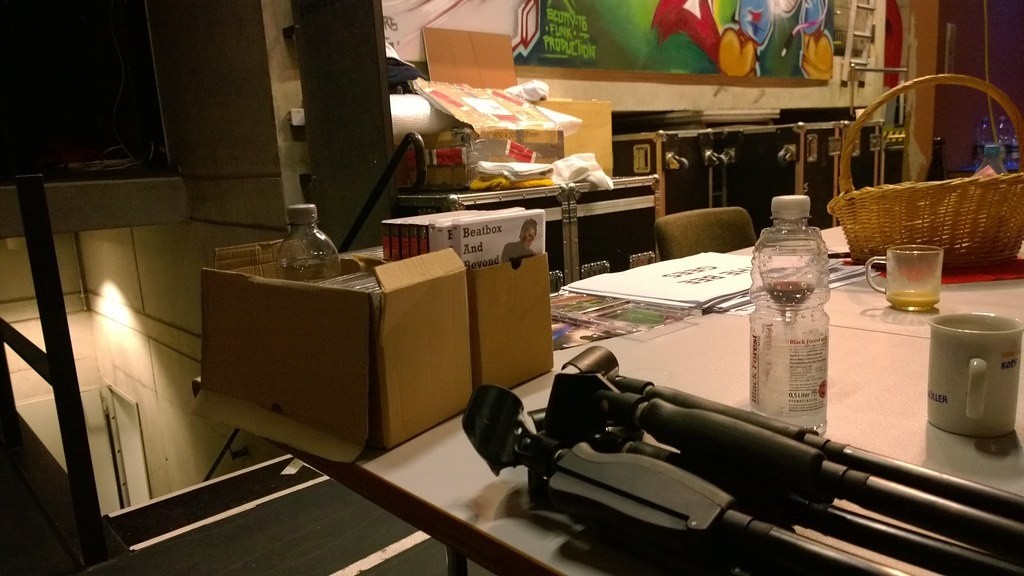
[750,195,830,435]
[277,204,341,282]
[996,116,1009,167]
[926,137,948,182]
[1004,127,1020,172]
[975,118,993,169]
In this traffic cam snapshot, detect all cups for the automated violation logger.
[867,245,944,311]
[926,313,1024,437]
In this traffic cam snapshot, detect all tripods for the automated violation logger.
[462,345,1024,576]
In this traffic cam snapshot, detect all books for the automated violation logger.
[451,211,546,268]
[379,205,546,269]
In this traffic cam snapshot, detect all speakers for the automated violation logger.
[0,0,170,187]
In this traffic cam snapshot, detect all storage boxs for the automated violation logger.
[567,174,660,281]
[397,128,565,190]
[399,186,571,294]
[613,127,718,221]
[352,252,554,388]
[189,239,473,463]
[719,121,886,236]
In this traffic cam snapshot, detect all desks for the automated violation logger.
[264,256,1024,576]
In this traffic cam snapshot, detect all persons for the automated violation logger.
[501,218,538,263]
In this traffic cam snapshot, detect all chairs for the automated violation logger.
[655,207,757,262]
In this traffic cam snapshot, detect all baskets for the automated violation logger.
[825,73,1024,266]
